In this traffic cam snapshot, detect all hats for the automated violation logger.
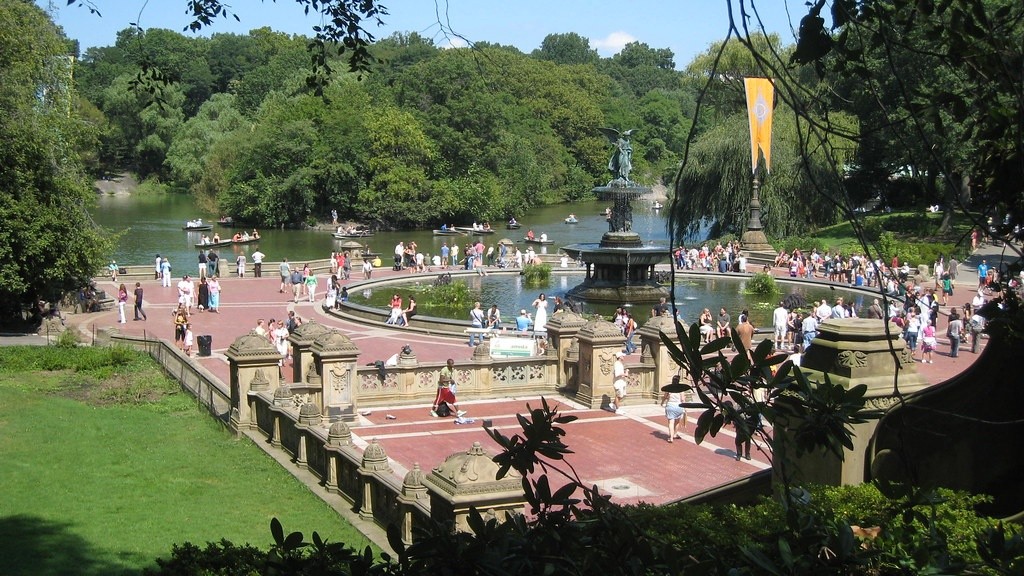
[616,351,626,358]
[521,309,526,313]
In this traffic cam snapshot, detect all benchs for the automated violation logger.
[97,291,115,312]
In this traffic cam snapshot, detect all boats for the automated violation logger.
[182,225,213,231]
[652,204,665,210]
[340,241,365,249]
[216,219,237,226]
[564,217,579,225]
[524,236,555,245]
[195,238,233,250]
[331,230,375,237]
[598,211,611,222]
[331,250,384,258]
[232,235,261,243]
[432,226,496,235]
[506,223,522,230]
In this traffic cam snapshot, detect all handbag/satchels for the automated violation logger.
[948,287,953,296]
[482,320,485,327]
[680,411,687,429]
[169,266,172,271]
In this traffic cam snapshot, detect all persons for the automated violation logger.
[108,201,1024,442]
[614,131,633,182]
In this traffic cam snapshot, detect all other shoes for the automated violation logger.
[615,408,625,415]
[673,434,681,439]
[667,438,673,443]
[929,360,933,364]
[144,315,147,321]
[736,456,740,461]
[922,359,927,364]
[746,454,751,460]
[134,318,139,321]
[608,403,616,410]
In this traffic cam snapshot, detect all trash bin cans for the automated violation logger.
[197,334,211,356]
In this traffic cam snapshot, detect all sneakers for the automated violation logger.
[456,410,466,417]
[430,410,438,418]
